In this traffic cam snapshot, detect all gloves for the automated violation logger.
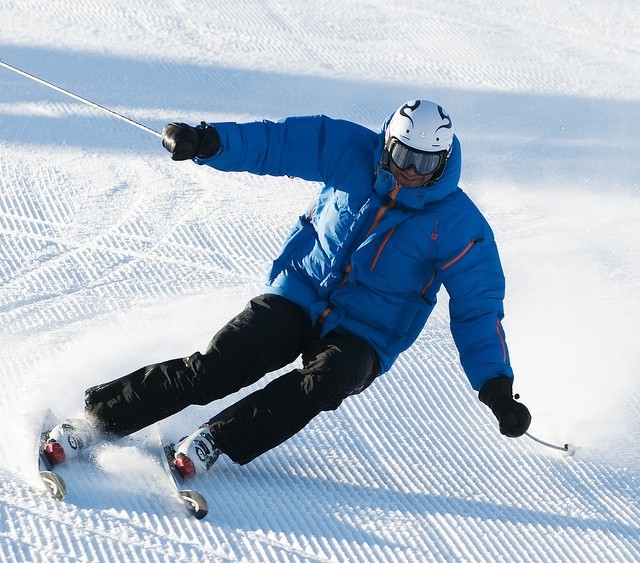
[160,121,220,162]
[477,379,531,438]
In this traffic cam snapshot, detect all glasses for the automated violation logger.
[386,135,450,176]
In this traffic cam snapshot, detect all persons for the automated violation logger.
[40,99,532,478]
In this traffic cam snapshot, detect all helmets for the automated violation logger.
[384,101,454,184]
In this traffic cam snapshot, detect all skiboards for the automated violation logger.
[39,405,208,521]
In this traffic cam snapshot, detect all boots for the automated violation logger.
[175,425,218,476]
[46,416,101,463]
